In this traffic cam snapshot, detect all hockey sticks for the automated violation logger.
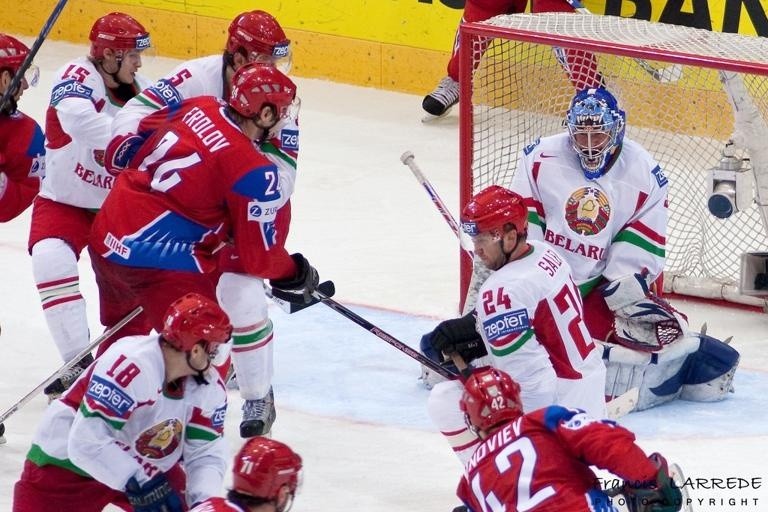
[561,1,700,88]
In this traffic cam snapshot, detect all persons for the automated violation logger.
[422,0,608,115]
[429,187,606,463]
[191,437,304,512]
[89,64,319,358]
[28,13,151,397]
[510,88,670,340]
[0,34,46,223]
[112,11,302,439]
[454,368,682,511]
[11,294,232,512]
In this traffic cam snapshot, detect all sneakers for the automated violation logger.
[419,75,460,123]
[42,351,94,405]
[238,386,277,440]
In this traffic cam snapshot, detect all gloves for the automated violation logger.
[429,313,477,351]
[624,453,684,512]
[269,252,322,307]
[126,472,185,512]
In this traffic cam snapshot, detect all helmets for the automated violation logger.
[564,88,628,161]
[224,9,292,66]
[0,34,34,83]
[161,292,235,362]
[228,60,297,123]
[458,365,525,434]
[89,12,151,62]
[457,183,530,242]
[232,437,303,510]
